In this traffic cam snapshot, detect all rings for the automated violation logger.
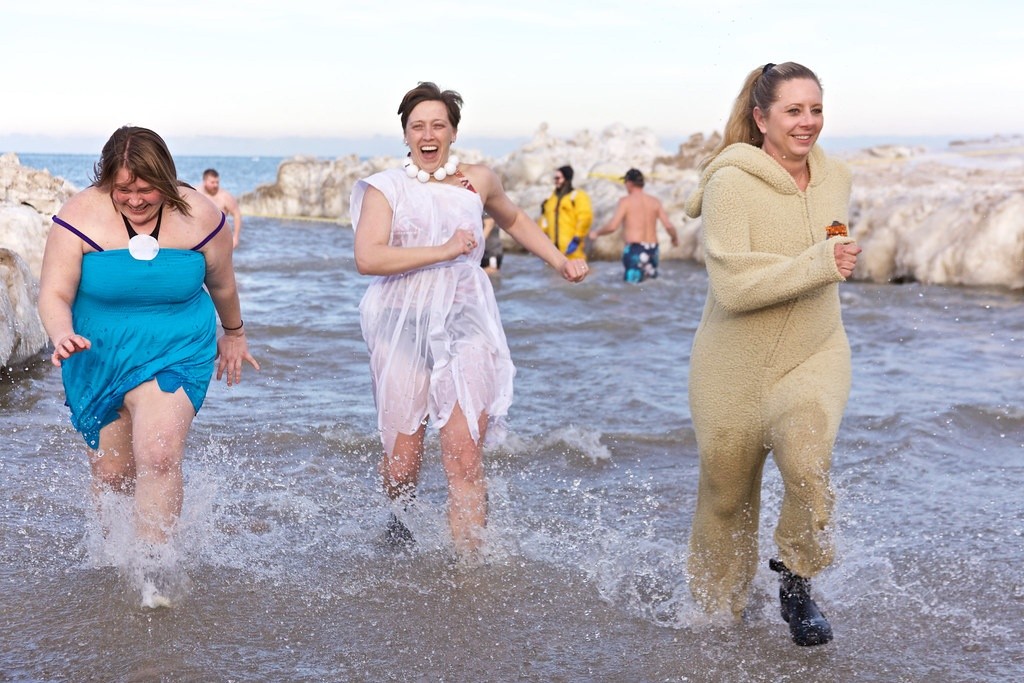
[466,240,471,248]
[579,265,584,269]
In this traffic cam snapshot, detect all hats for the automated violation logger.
[619,168,642,179]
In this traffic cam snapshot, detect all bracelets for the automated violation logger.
[220,320,244,330]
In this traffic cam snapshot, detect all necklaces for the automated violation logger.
[403,149,462,182]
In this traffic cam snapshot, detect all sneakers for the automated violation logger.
[769,557,835,646]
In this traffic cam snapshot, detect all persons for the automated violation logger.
[587,168,680,286]
[532,164,594,282]
[35,123,262,614]
[347,80,591,573]
[193,167,242,249]
[684,57,862,646]
[475,203,505,275]
